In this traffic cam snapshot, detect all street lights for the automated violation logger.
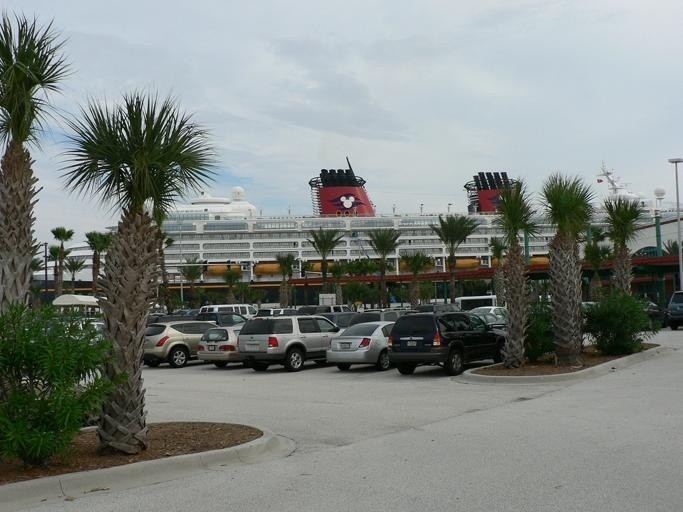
[668,158,683,291]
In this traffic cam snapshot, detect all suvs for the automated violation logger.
[143,321,221,368]
[388,310,506,376]
[237,315,347,372]
[667,291,683,329]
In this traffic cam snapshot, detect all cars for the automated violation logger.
[147,295,552,331]
[197,326,253,368]
[326,321,396,371]
[63,322,107,345]
